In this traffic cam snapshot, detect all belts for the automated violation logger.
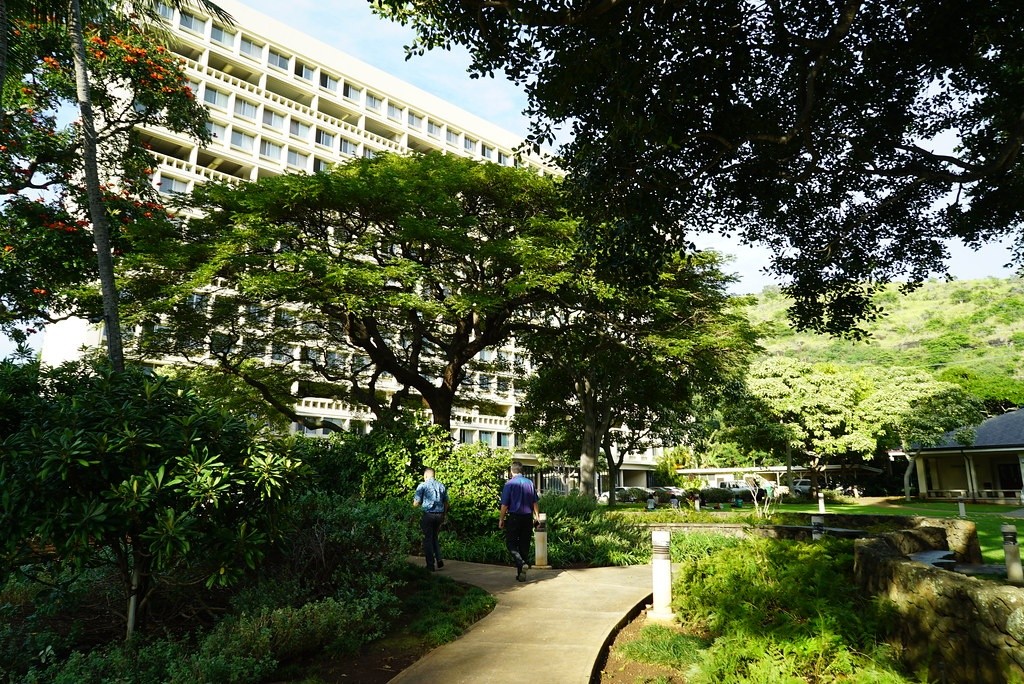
[430,512,443,514]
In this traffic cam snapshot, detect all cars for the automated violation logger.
[793,479,813,497]
[650,486,684,496]
[601,486,631,502]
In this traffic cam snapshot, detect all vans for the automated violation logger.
[719,480,750,492]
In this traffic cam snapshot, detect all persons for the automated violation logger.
[686,491,707,508]
[498,462,541,582]
[646,494,657,510]
[668,494,679,508]
[413,468,449,572]
[652,492,662,506]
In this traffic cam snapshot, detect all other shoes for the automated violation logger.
[518,563,529,582]
[425,566,434,572]
[437,561,443,567]
[516,576,519,580]
